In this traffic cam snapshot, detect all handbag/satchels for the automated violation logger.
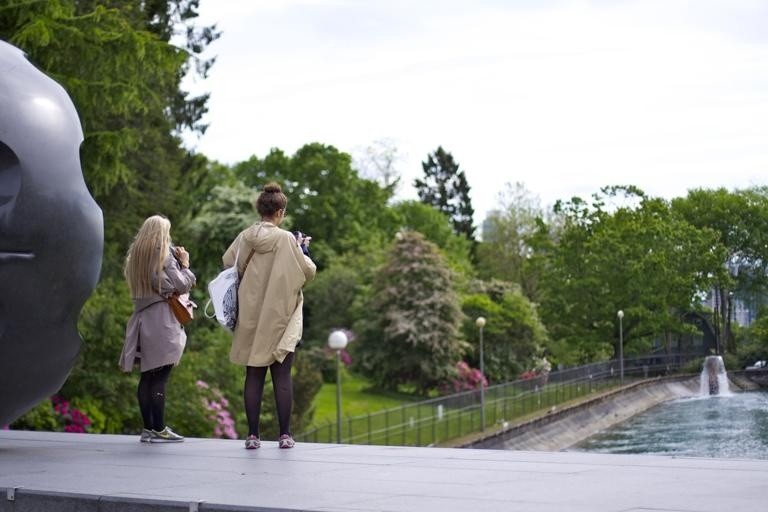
[208,266,239,331]
[170,292,198,326]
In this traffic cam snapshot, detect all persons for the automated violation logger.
[222,182,318,450]
[118,215,197,445]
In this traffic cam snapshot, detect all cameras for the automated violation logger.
[294,230,308,245]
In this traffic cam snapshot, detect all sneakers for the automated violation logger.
[140,426,185,443]
[246,434,261,449]
[279,434,295,448]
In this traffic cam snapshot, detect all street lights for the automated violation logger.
[328,331,349,444]
[476,316,487,431]
[617,310,624,383]
